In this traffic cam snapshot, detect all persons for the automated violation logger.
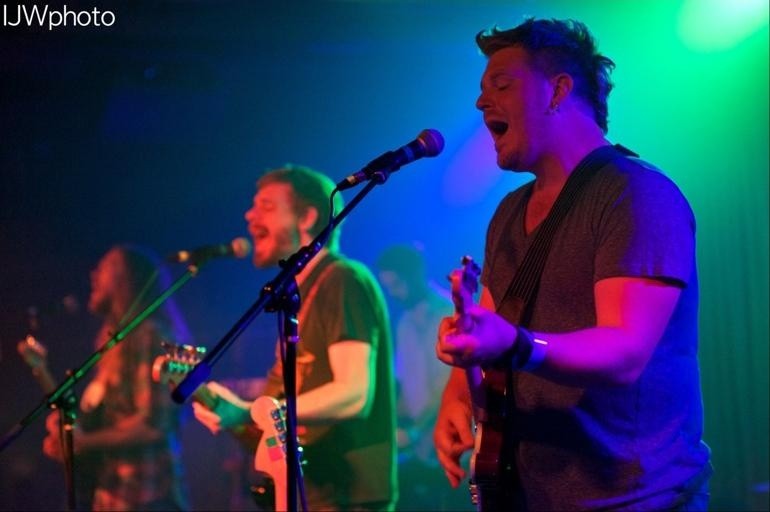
[191,164,398,512]
[47,244,181,511]
[434,18,712,511]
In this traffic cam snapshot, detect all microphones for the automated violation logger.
[335,129,445,192]
[168,238,250,263]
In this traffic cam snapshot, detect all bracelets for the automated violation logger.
[509,327,535,372]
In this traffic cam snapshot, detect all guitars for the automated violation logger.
[154,344,262,441]
[15,336,105,479]
[445,253,522,512]
[251,396,308,512]
